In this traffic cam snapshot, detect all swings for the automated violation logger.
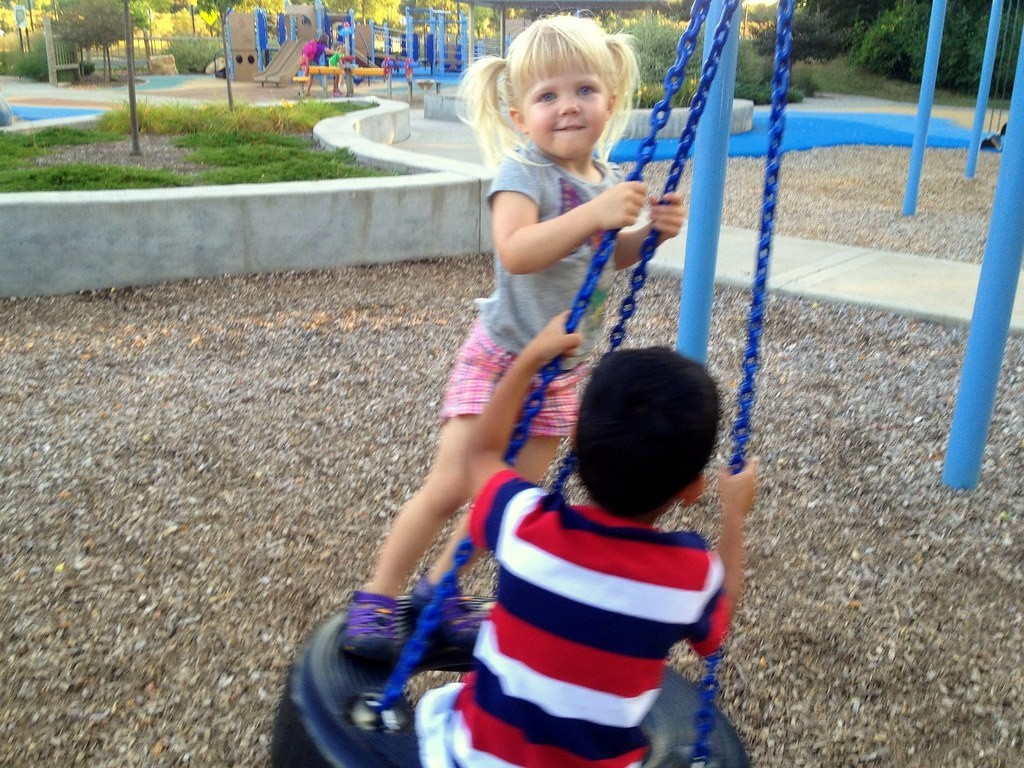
[981,0,1020,150]
[266,0,797,768]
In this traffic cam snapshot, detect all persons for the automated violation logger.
[300,33,347,95]
[402,309,757,767]
[344,16,683,673]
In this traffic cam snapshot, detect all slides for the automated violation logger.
[252,39,309,85]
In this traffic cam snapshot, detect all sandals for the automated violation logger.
[410,576,482,644]
[346,583,396,652]
